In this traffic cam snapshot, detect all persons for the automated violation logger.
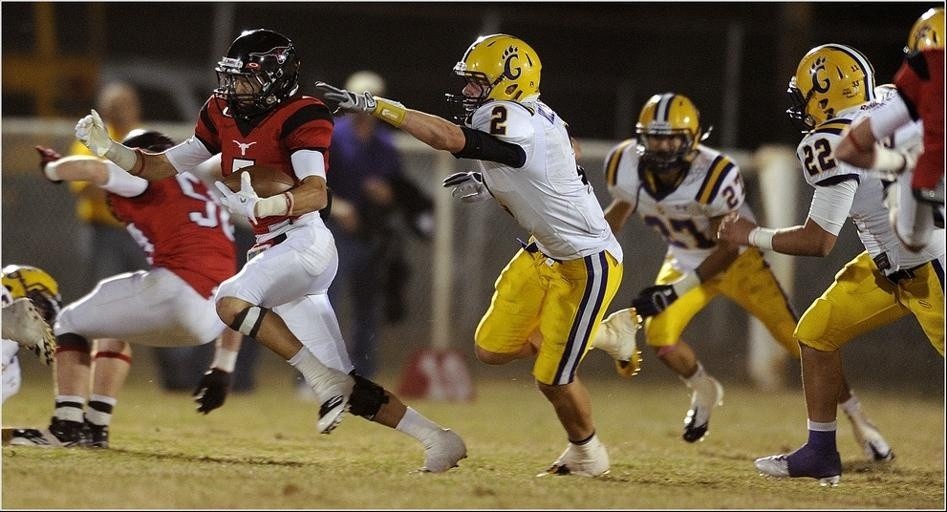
[313,30,643,478]
[717,43,944,484]
[2,260,63,415]
[6,128,249,450]
[828,8,946,258]
[75,27,469,472]
[602,91,895,462]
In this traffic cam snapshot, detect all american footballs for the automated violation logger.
[223,167,295,196]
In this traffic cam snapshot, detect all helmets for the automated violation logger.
[2,262,64,323]
[633,92,702,173]
[786,43,876,135]
[907,7,944,53]
[119,128,176,151]
[213,28,301,121]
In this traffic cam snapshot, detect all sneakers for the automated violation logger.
[682,376,725,442]
[755,444,842,488]
[854,421,895,463]
[408,429,468,475]
[10,415,84,449]
[82,412,109,449]
[316,369,359,435]
[538,444,610,479]
[4,297,58,367]
[603,308,644,377]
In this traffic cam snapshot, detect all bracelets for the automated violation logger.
[132,148,144,178]
[255,190,295,220]
[369,94,407,133]
[745,224,774,250]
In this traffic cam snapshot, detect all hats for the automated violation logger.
[444,32,542,123]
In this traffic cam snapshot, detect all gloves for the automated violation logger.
[215,171,262,225]
[75,109,115,157]
[192,366,237,416]
[315,80,377,118]
[443,170,494,203]
[36,146,65,184]
[631,284,679,321]
